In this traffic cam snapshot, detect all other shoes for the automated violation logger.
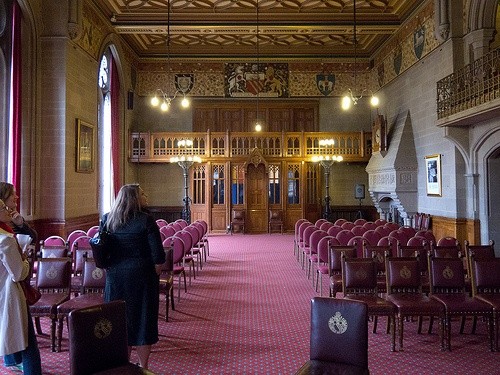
[5,362,24,373]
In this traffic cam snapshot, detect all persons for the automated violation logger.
[100,184,166,375]
[0,180,37,371]
[0,199,42,375]
[428,165,436,182]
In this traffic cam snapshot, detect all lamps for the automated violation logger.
[150,0,191,115]
[340,0,380,111]
[252,0,263,132]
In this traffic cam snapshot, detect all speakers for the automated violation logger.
[354,184,365,199]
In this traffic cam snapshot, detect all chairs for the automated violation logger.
[269,210,283,236]
[294,217,500,375]
[23,219,209,375]
[230,208,246,236]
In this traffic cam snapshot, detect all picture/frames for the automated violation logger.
[424,154,443,197]
[75,119,95,174]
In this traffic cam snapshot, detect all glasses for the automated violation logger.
[0,205,9,211]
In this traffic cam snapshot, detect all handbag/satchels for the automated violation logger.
[20,280,42,305]
[88,220,122,270]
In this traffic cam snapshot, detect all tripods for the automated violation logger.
[352,199,366,223]
